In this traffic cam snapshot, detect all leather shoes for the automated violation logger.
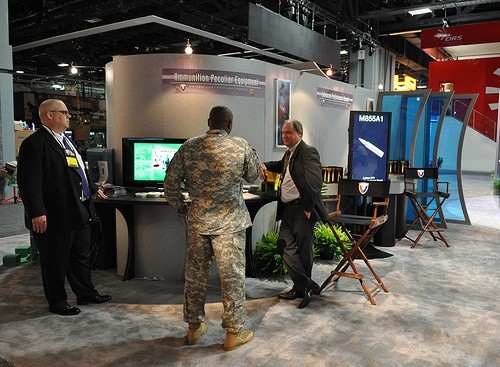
[76,293,112,305]
[49,304,81,315]
[298,279,320,309]
[278,285,305,300]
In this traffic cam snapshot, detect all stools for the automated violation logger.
[314,177,392,305]
[399,167,450,248]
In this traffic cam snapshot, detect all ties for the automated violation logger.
[63,137,91,198]
[279,150,291,197]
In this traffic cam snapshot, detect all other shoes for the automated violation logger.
[188,323,207,345]
[223,328,254,351]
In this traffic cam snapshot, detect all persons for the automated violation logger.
[16,98,112,316]
[259,120,330,309]
[164,106,260,351]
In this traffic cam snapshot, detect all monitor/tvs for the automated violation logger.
[121,138,188,192]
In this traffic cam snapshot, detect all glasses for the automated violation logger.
[50,110,68,115]
